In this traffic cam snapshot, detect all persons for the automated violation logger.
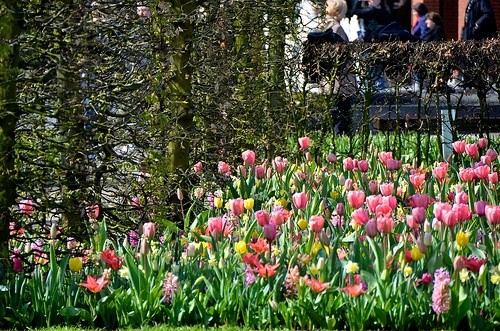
[420,13,443,42]
[460,0,494,42]
[324,0,357,95]
[351,0,405,90]
[411,3,430,39]
